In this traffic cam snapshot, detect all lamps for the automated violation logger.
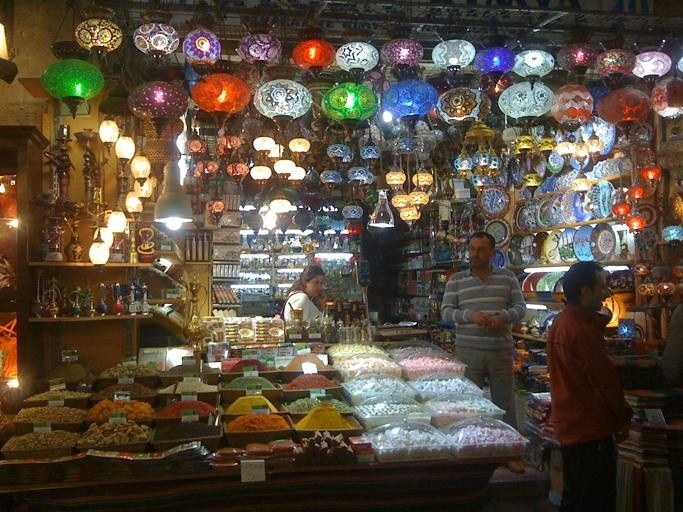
[43,3,682,274]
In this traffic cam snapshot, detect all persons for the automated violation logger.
[440,231,527,473]
[661,303,682,386]
[545,260,635,512]
[283,265,326,322]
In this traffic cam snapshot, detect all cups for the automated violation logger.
[290,311,303,319]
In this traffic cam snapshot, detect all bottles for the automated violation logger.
[320,300,366,343]
[240,234,352,271]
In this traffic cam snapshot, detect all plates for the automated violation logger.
[478,117,618,337]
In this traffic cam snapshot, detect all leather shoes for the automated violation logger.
[507,461,525,473]
